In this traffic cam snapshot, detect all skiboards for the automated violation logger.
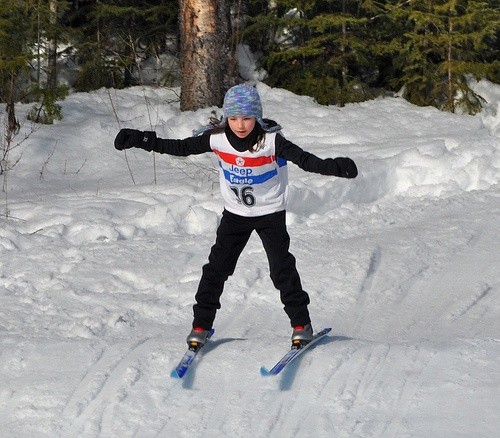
[170,327,334,379]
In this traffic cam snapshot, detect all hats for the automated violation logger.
[192,83,282,137]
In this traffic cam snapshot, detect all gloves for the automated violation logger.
[320,157,358,178]
[114,128,156,152]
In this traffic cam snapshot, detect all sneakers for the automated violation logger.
[187,327,208,345]
[291,324,313,341]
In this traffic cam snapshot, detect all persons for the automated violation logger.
[114,83,358,348]
